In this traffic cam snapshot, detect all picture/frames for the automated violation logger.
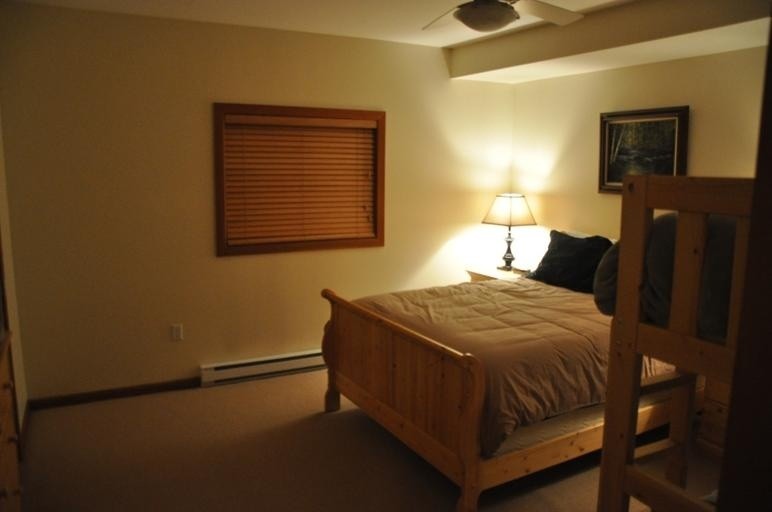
[598,105,689,194]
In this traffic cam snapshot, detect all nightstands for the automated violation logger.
[468,267,531,284]
[694,375,733,464]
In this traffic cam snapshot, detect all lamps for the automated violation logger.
[482,193,536,270]
[453,0,520,33]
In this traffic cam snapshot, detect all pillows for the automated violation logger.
[525,231,612,294]
[593,213,735,336]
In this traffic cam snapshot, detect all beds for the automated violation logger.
[320,272,708,512]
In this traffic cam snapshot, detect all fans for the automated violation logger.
[421,1,584,32]
